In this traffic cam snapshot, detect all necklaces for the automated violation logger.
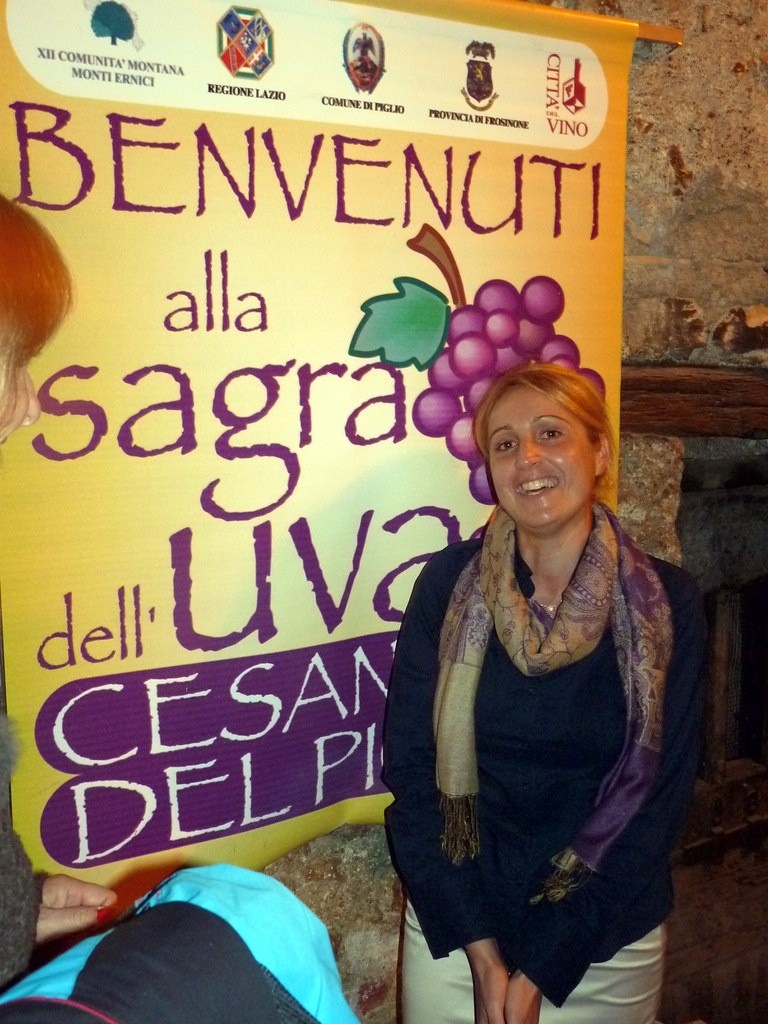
[530,589,564,612]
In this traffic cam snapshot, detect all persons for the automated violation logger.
[0,196,121,1020]
[380,362,718,1023]
